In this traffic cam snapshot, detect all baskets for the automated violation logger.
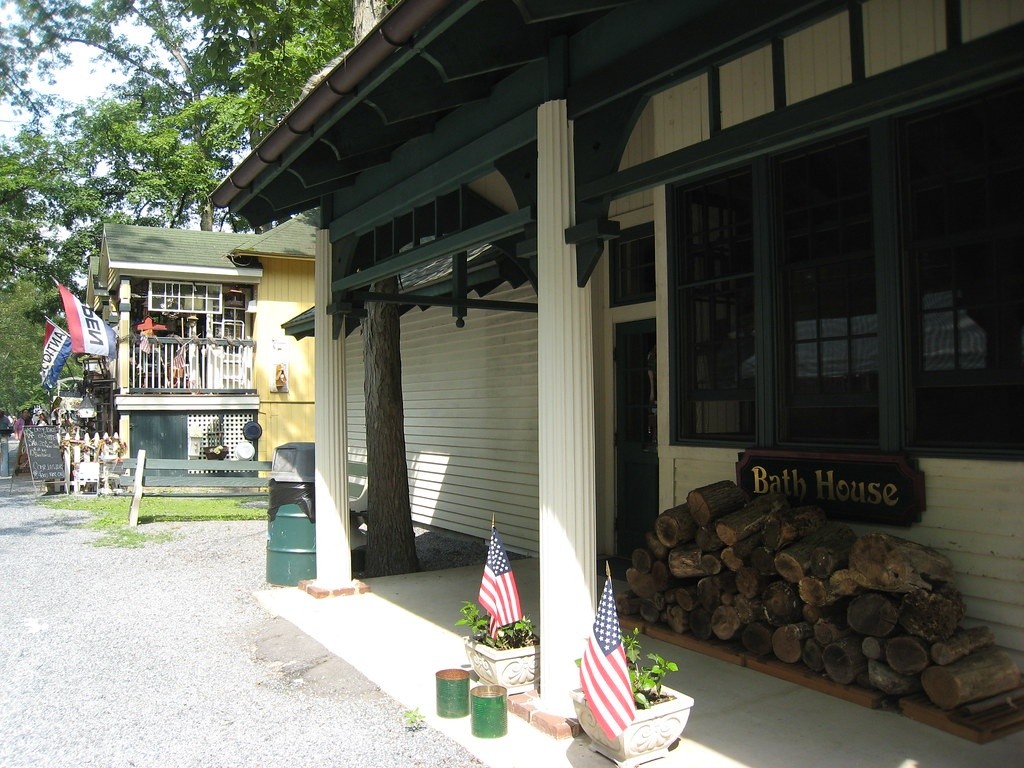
[203,446,228,460]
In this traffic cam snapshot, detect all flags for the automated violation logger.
[58,285,117,359]
[479,526,522,640]
[580,579,635,739]
[42,321,72,390]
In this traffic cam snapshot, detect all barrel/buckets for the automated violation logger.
[267,479,316,586]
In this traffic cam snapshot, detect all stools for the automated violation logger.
[0,476,13,496]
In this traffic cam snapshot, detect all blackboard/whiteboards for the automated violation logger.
[22,425,65,479]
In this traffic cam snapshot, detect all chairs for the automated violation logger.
[77,462,100,496]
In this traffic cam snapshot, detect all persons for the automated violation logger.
[0,409,49,440]
[161,331,178,387]
[645,351,656,440]
[85,422,97,439]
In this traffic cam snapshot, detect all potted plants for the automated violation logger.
[453,600,540,697]
[571,627,694,768]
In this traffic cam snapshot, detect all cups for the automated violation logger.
[436,669,470,719]
[470,685,507,738]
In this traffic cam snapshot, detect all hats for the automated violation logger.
[235,442,255,461]
[243,422,263,440]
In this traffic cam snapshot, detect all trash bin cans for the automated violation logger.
[265,441,317,586]
